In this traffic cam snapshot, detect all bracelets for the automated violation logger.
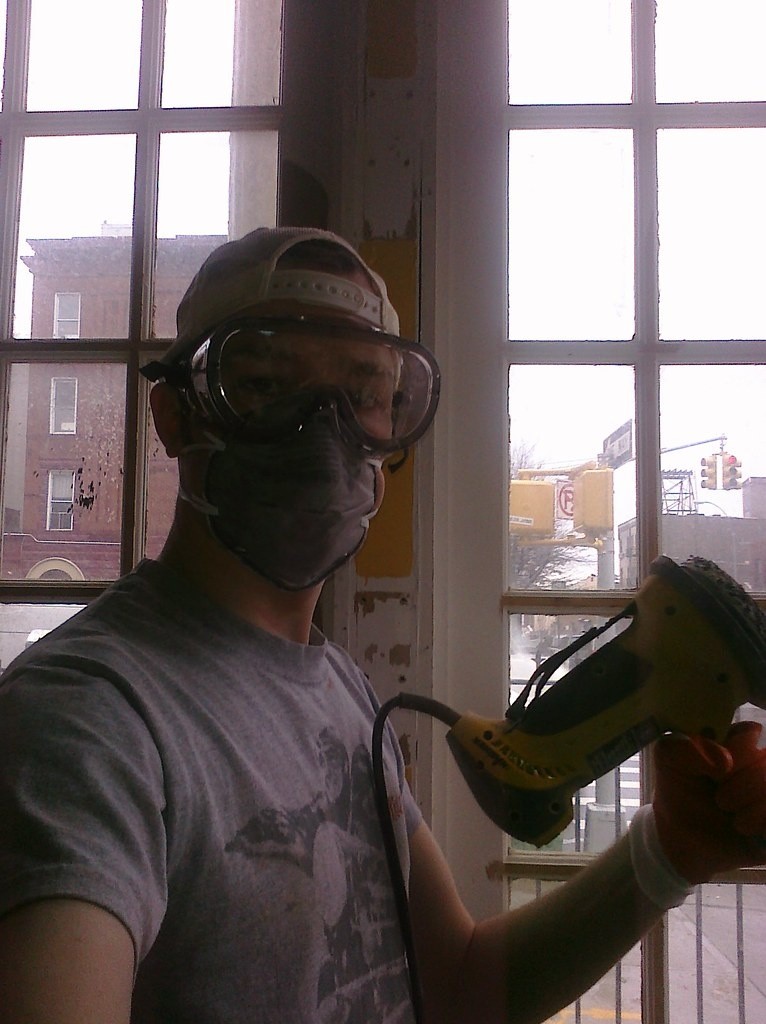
[629,805,696,911]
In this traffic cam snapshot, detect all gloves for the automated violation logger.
[628,719,766,910]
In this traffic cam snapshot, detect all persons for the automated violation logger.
[0,226,766,1024]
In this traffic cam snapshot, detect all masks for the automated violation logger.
[199,420,385,594]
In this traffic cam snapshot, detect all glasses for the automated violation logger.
[163,315,442,454]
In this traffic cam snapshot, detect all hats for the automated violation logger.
[161,222,400,365]
